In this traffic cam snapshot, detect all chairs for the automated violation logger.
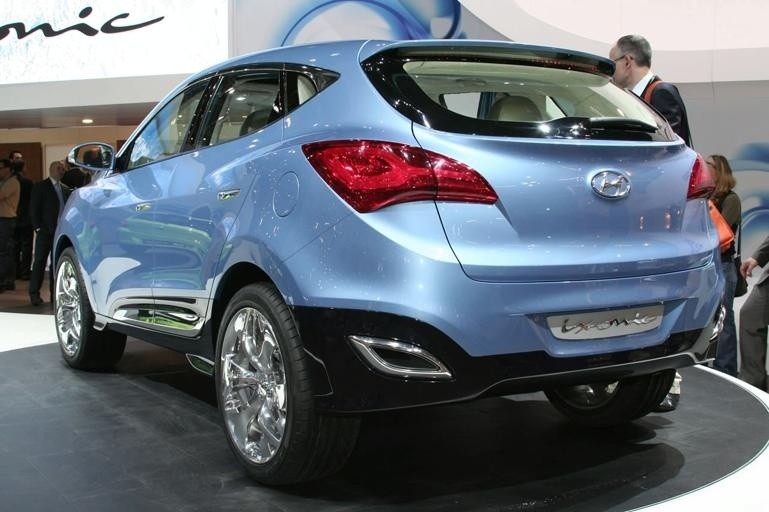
[485,95,543,121]
[240,109,272,136]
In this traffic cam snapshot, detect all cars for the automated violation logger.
[71,216,103,270]
[112,207,231,269]
[50,29,728,483]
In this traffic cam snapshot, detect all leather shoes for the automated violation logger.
[653,392,681,411]
[16,271,32,281]
[0,279,15,290]
[31,294,43,306]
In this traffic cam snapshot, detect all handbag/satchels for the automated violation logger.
[733,256,750,297]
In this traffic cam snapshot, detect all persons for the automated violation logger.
[608,34,690,413]
[705,155,741,377]
[739,236,768,393]
[0,150,95,306]
